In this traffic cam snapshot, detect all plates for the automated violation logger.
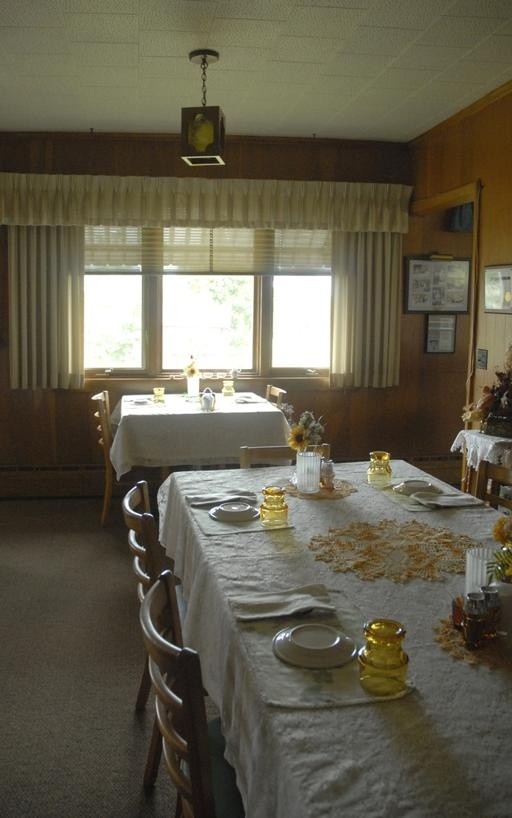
[208,501,260,523]
[391,480,439,496]
[272,622,358,669]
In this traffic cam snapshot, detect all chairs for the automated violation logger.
[475,460,511,513]
[89,384,293,528]
[134,569,247,818]
[119,479,172,721]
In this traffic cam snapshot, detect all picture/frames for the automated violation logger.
[401,256,512,354]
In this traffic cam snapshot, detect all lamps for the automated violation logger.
[181,48,226,167]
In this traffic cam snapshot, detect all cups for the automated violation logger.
[465,547,502,601]
[296,451,322,494]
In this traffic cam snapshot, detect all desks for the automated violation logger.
[153,456,511,816]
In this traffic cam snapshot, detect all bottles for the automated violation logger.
[151,387,165,404]
[259,485,289,529]
[357,618,410,694]
[221,380,236,396]
[450,584,500,648]
[366,450,393,489]
[320,455,335,490]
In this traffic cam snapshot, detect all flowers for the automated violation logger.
[183,353,202,377]
[287,410,327,452]
